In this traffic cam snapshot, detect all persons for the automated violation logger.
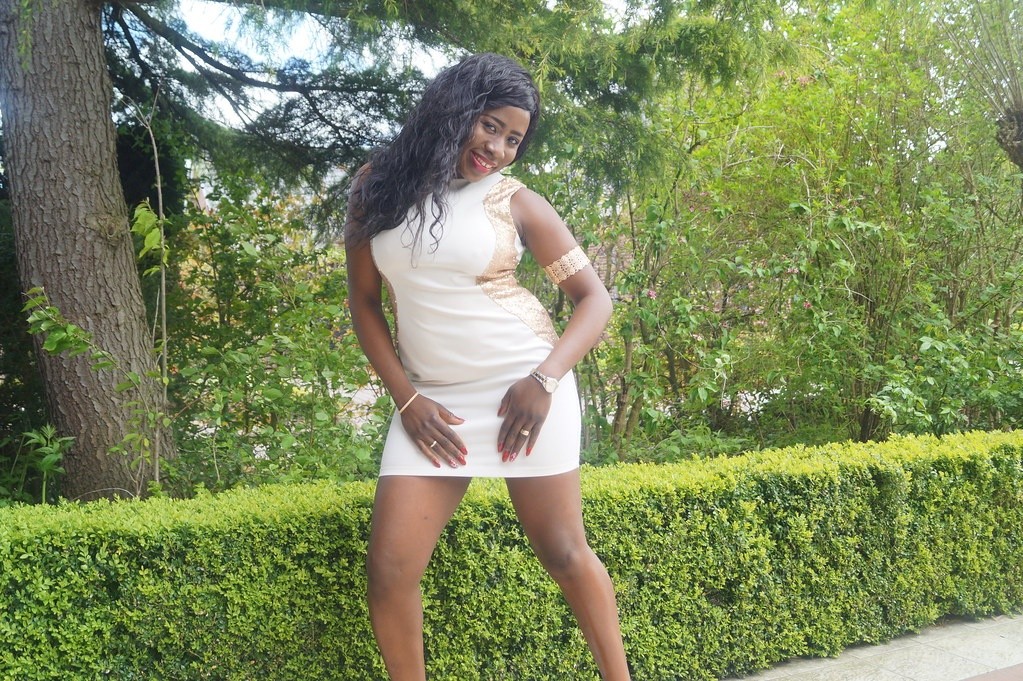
[344,53,631,681]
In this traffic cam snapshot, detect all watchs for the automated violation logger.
[531,368,559,393]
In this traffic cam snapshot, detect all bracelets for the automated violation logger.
[399,393,419,414]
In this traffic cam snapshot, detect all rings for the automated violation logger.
[520,429,529,435]
[431,441,437,447]
